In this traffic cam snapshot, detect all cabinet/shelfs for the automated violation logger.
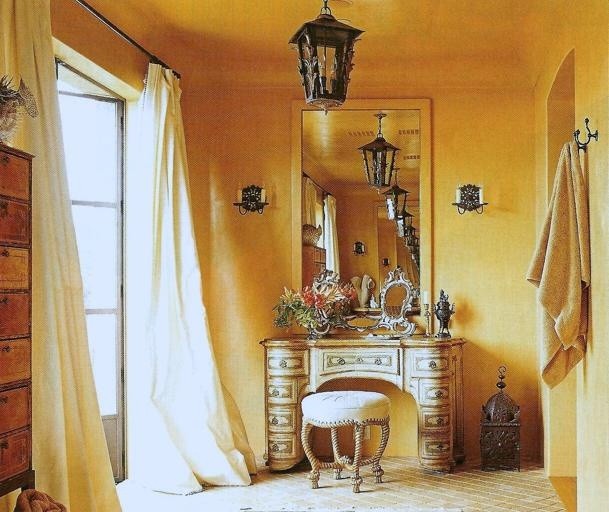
[302,245,326,292]
[0,143,35,500]
[259,337,466,473]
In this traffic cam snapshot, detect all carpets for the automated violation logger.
[116,454,570,512]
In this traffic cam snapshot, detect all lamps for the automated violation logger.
[382,167,410,221]
[351,240,368,257]
[232,182,269,214]
[287,0,365,116]
[451,185,488,215]
[395,210,419,273]
[356,110,401,195]
[481,365,522,472]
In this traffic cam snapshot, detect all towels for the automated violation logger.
[13,488,67,512]
[525,142,590,391]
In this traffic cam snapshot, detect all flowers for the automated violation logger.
[271,271,361,330]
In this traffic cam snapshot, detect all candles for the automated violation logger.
[422,289,429,305]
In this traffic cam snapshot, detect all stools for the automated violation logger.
[300,391,391,494]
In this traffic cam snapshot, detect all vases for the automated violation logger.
[304,322,332,342]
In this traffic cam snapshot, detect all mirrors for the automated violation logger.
[370,265,420,341]
[289,99,434,337]
